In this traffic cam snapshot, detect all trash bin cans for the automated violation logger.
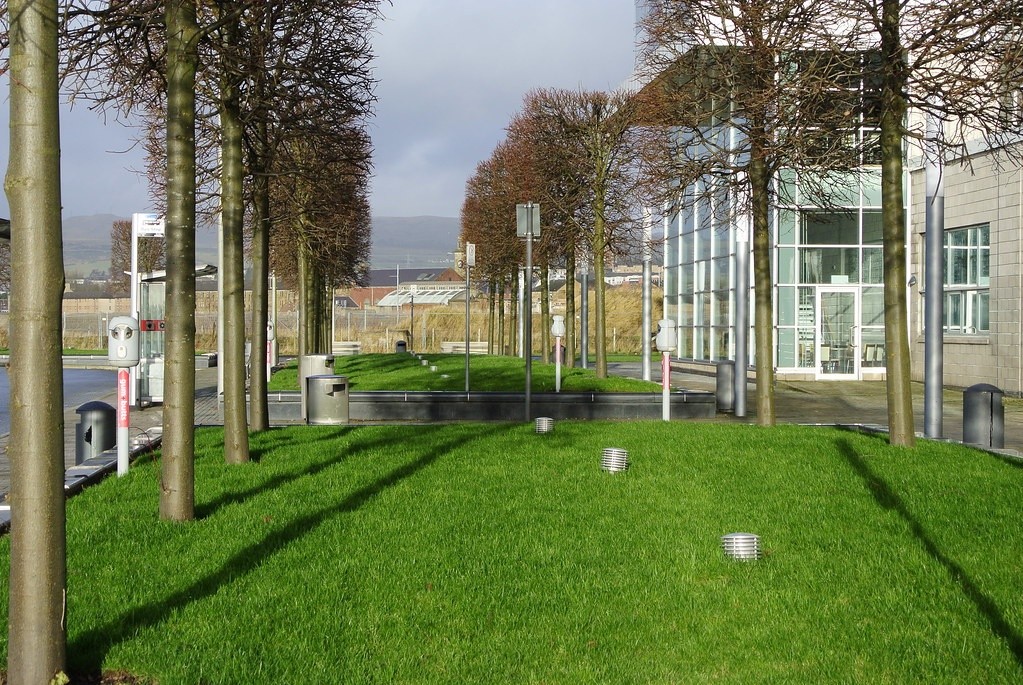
[75,401,116,465]
[396,341,406,353]
[300,354,335,419]
[305,375,349,425]
[963,383,1004,449]
[552,344,565,364]
[717,360,736,413]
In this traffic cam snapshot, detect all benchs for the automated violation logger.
[441,342,499,355]
[331,341,361,355]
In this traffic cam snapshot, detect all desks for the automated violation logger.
[830,348,851,373]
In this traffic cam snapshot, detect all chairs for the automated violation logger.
[799,343,885,374]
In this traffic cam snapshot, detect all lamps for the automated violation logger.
[422,360,429,365]
[429,365,438,372]
[599,447,629,475]
[908,276,916,285]
[721,532,762,562]
[534,417,554,434]
